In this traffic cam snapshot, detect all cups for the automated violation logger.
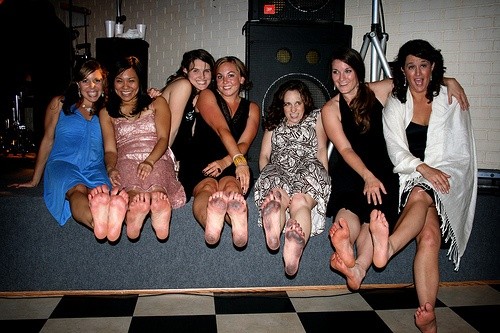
[115,23,124,37]
[105,20,115,38]
[136,24,147,40]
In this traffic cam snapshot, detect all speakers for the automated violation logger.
[249,0,345,24]
[245,20,354,181]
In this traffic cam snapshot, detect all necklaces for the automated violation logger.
[286,120,298,126]
[81,103,95,115]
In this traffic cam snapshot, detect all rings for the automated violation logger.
[435,180,439,185]
[217,168,221,174]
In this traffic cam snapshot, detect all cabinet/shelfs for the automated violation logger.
[96,38,149,97]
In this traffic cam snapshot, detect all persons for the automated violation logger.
[160,48,216,202]
[369,39,478,333]
[6,58,163,243]
[321,48,471,291]
[253,79,331,278]
[191,55,260,247]
[99,56,187,239]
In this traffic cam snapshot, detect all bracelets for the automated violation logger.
[232,154,247,165]
